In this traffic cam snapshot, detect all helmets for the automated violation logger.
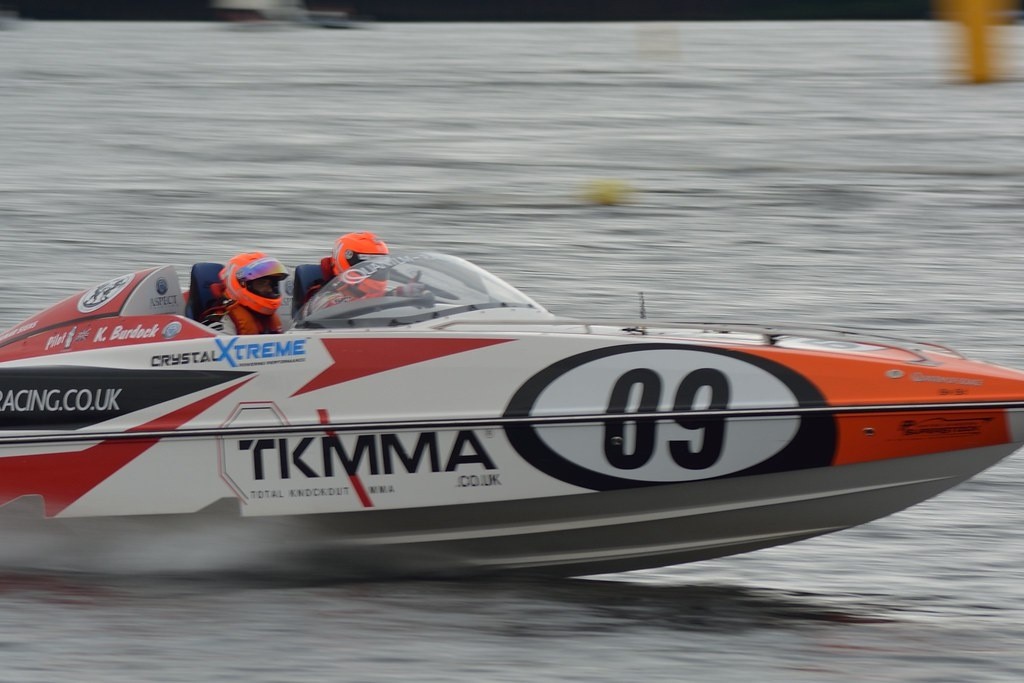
[222,252,289,316]
[330,231,391,297]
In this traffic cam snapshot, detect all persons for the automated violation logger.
[201,252,289,335]
[299,233,425,320]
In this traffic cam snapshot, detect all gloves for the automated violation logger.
[393,282,427,298]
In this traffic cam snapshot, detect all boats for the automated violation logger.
[0,250,1020,573]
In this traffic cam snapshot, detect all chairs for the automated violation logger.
[184,262,226,324]
[292,264,322,328]
